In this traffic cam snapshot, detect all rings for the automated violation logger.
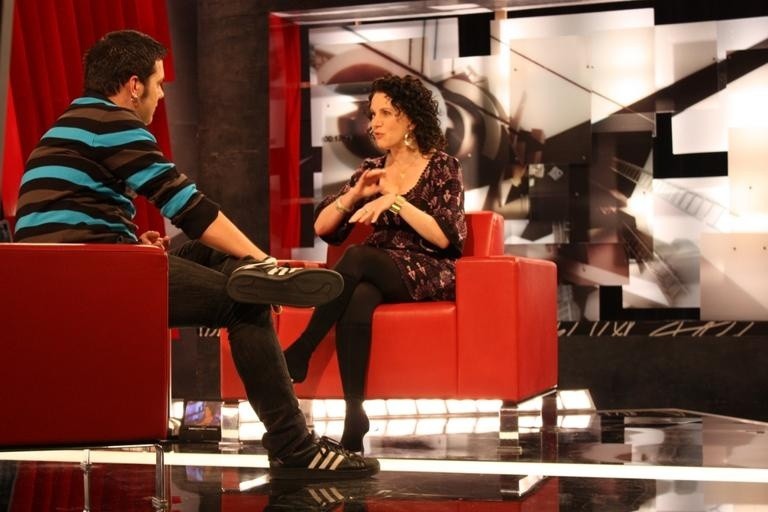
[363,208,369,213]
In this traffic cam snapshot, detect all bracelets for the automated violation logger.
[262,256,278,265]
[387,194,407,217]
[332,194,354,217]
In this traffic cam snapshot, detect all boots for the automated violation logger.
[338,397,370,452]
[282,332,317,384]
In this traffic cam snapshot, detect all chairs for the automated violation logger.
[0,241,172,470]
[218,210,562,435]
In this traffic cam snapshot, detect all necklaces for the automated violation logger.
[389,145,421,182]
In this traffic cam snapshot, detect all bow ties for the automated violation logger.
[266,428,381,481]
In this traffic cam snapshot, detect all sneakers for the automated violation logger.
[225,253,346,308]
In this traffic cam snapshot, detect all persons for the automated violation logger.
[10,29,382,482]
[262,476,382,512]
[280,72,468,454]
